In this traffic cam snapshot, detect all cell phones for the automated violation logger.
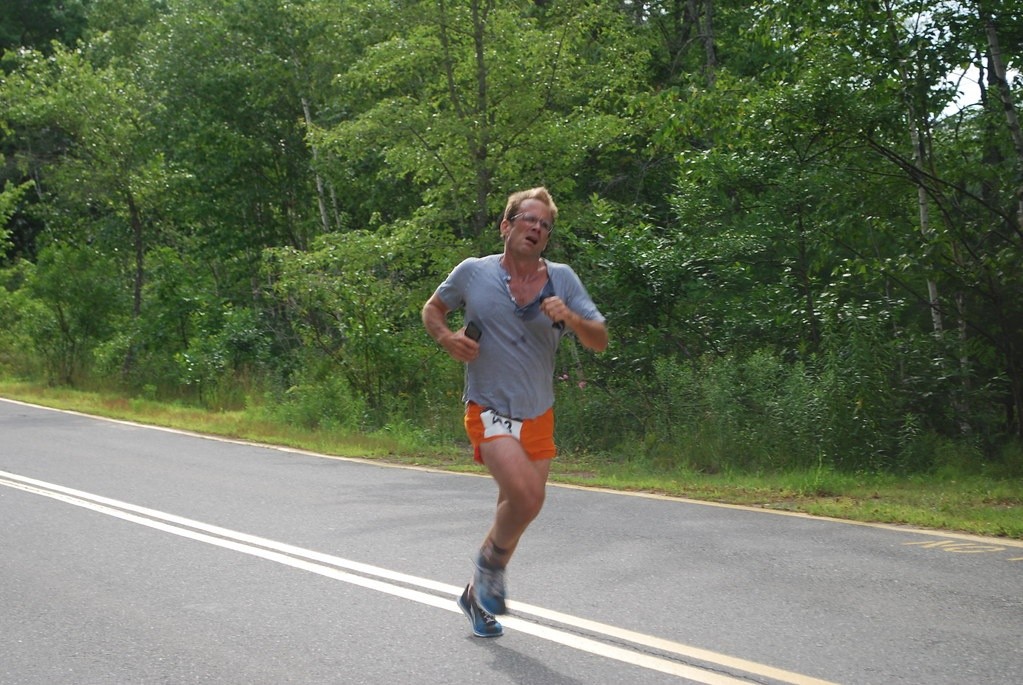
[464,321,482,342]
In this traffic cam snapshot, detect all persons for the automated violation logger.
[422,188,608,637]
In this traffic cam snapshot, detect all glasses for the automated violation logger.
[510,212,555,235]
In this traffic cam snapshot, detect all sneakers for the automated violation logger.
[473,549,507,617]
[457,583,504,638]
